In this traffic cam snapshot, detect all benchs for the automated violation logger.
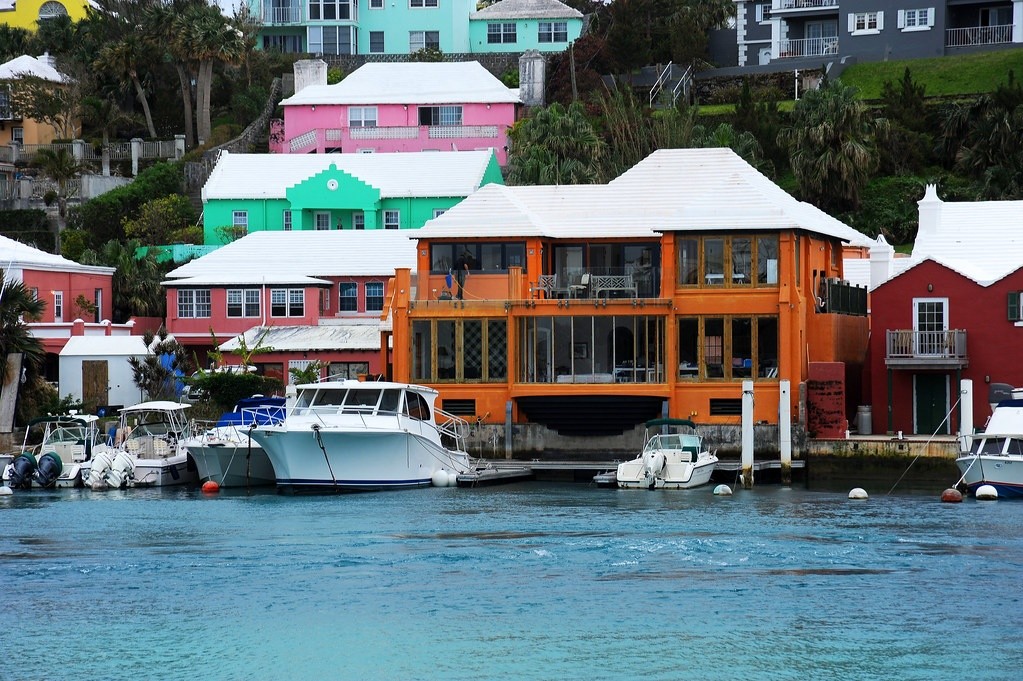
[590,274,638,299]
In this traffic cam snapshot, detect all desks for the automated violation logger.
[704,273,745,283]
[613,367,698,382]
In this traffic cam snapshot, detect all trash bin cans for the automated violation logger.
[238,398,286,412]
[858,405,872,434]
[96,405,124,417]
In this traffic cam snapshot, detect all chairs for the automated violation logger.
[530,274,557,299]
[570,274,590,299]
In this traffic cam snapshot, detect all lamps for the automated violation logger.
[311,104,316,113]
[404,104,408,111]
[486,104,491,109]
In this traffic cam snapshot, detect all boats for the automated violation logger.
[235,370,470,495]
[112,398,199,491]
[953,387,1023,501]
[181,394,287,492]
[616,416,720,491]
[455,462,532,490]
[592,467,618,487]
[25,408,104,487]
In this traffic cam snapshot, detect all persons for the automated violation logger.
[637,353,652,382]
[455,250,470,300]
